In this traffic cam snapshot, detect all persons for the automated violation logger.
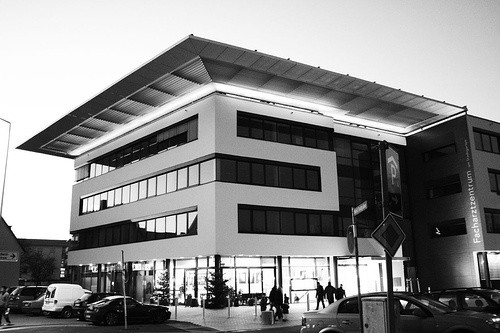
[260,296,270,316]
[281,300,290,321]
[272,286,286,322]
[335,283,346,301]
[0,285,12,325]
[269,285,277,311]
[284,293,290,304]
[315,281,325,310]
[323,281,336,305]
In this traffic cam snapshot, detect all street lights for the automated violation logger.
[0,118,11,216]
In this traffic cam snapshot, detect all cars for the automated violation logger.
[6,283,171,326]
[299,290,500,333]
[404,286,500,319]
